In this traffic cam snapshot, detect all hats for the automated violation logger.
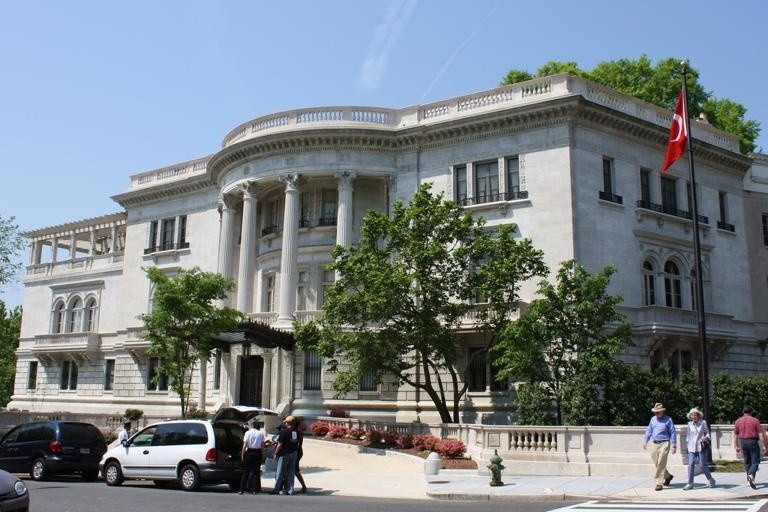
[687,408,703,419]
[124,422,131,426]
[283,416,294,423]
[652,402,665,412]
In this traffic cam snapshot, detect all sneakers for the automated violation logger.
[268,488,305,494]
[747,475,756,489]
[709,479,715,488]
[683,483,693,490]
[664,475,673,485]
[655,484,662,491]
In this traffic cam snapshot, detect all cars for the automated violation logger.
[0,470,29,512]
[0,421,108,480]
[99,406,279,491]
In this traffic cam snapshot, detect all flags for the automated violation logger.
[663,93,687,174]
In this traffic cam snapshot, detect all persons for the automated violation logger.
[733,406,767,489]
[643,403,676,491]
[238,416,306,495]
[682,407,715,490]
[119,422,131,444]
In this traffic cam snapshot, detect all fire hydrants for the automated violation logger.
[487,450,506,486]
